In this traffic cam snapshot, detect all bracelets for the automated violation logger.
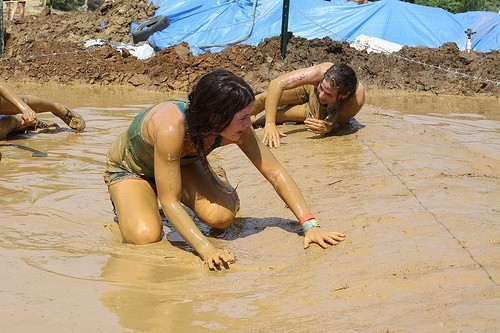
[301,219,322,232]
[299,212,317,225]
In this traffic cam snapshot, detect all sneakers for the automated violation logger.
[51,105,86,130]
[34,120,60,130]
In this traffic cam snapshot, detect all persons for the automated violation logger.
[5,2,10,21]
[11,0,26,22]
[0,80,86,139]
[249,62,366,148]
[104,68,346,271]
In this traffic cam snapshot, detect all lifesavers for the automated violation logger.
[133,15,170,44]
